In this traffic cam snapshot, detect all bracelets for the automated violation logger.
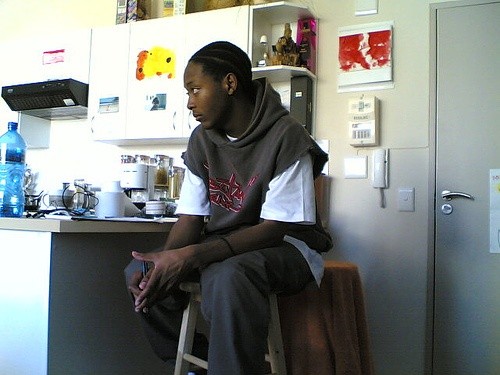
[219,237,238,257]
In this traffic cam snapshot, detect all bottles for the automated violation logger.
[95,191,124,218]
[257,34,269,67]
[0,122,26,219]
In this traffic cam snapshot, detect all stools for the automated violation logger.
[277,259,374,375]
[170,276,292,375]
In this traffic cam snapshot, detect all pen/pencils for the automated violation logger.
[142,259,148,278]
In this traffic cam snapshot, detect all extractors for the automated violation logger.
[2,28,90,123]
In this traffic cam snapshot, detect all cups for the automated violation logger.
[43,190,74,211]
[24,191,44,212]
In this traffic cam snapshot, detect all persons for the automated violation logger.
[122,40,334,375]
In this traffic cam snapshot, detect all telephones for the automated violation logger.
[371,147,389,188]
